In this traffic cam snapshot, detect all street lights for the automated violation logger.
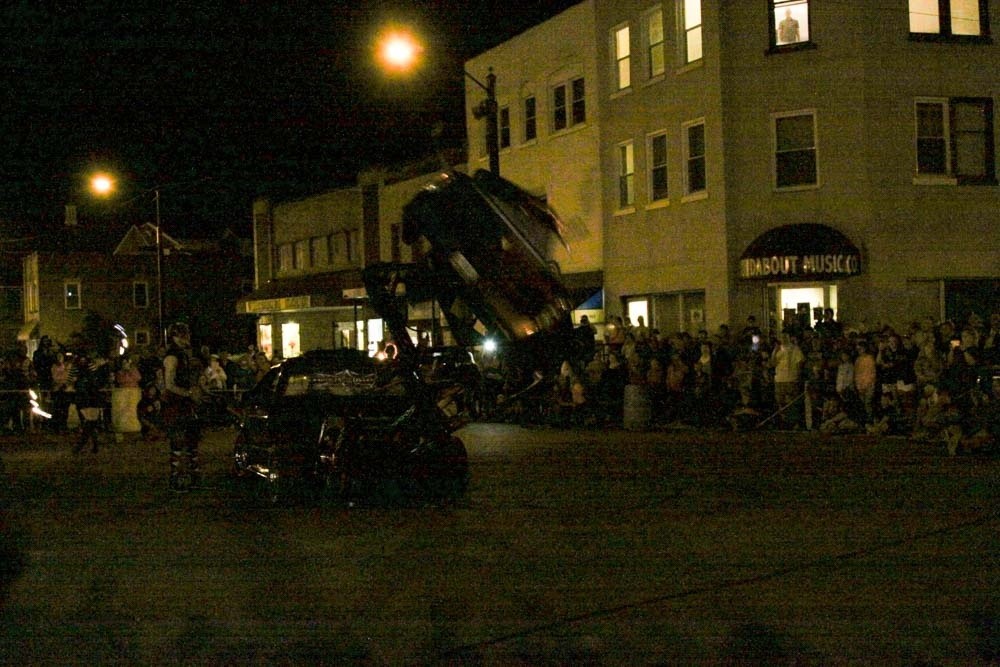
[373,21,499,176]
[87,171,166,348]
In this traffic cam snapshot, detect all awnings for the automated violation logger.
[236,270,379,315]
[739,223,863,283]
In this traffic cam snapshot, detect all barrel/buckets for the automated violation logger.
[624,385,656,430]
[111,387,143,434]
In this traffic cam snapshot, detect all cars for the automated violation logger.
[230,346,469,504]
[401,170,573,349]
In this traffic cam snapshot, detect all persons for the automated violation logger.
[2,330,286,437]
[556,304,1000,457]
[162,322,205,474]
[67,313,119,460]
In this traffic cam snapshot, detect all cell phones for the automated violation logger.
[950,341,960,346]
